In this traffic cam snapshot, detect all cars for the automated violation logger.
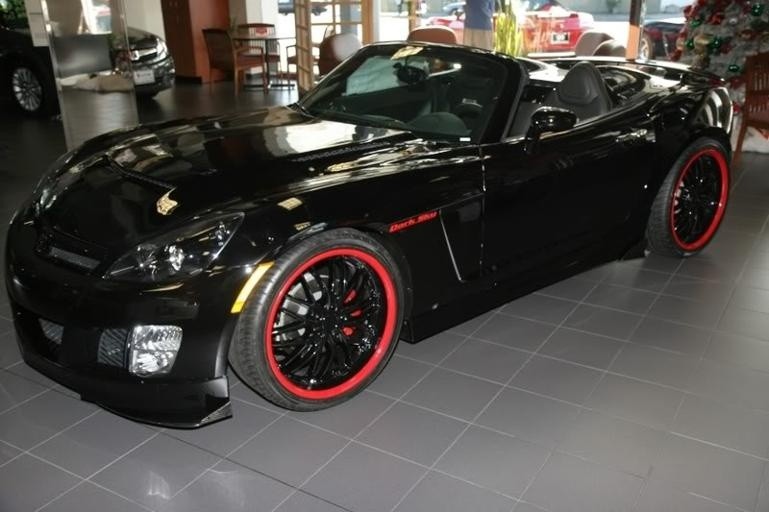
[0,9,177,118]
[428,0,596,56]
[4,40,733,430]
[640,16,689,61]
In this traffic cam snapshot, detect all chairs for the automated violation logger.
[318,34,361,74]
[509,61,613,141]
[455,64,503,118]
[574,31,626,56]
[406,25,456,44]
[201,23,335,96]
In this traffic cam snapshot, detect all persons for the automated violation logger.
[463,0,494,52]
